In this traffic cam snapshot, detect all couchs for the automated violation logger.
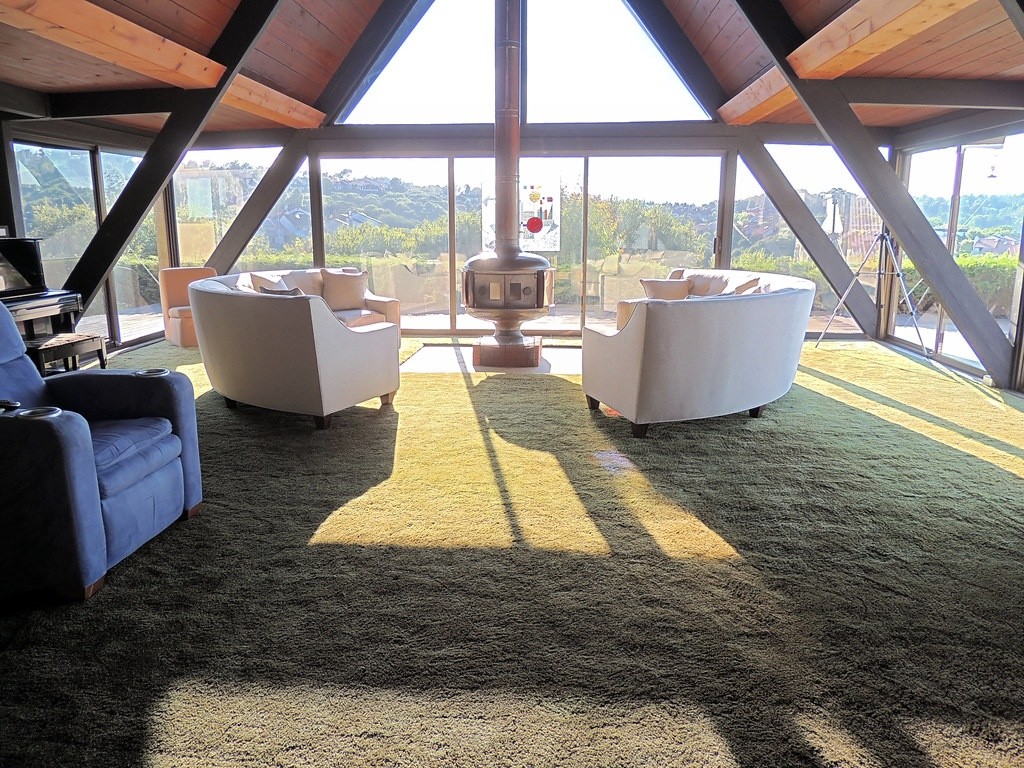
[158,266,217,348]
[580,268,816,440]
[0,301,207,602]
[188,269,402,429]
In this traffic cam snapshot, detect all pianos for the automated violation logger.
[0,237,108,378]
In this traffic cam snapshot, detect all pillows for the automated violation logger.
[572,250,698,310]
[251,272,288,290]
[320,268,370,310]
[259,285,304,296]
[361,251,469,318]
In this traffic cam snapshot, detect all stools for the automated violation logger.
[639,278,688,301]
[734,277,760,293]
[22,332,107,378]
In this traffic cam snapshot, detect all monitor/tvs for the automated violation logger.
[0,242,44,296]
